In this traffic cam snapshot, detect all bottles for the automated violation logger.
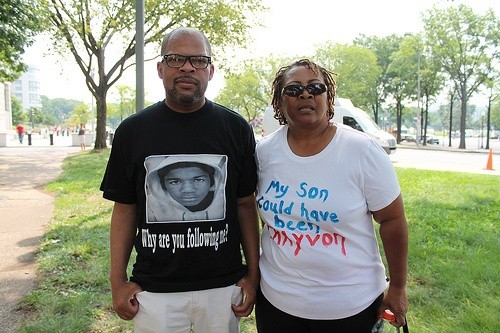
[372,309,400,333]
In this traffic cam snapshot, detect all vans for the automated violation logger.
[262,96,399,151]
[392,130,412,141]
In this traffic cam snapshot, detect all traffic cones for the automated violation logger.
[482,148,495,171]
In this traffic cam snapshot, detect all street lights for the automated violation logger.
[404,33,421,145]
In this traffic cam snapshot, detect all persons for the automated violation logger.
[147,156,225,220]
[17,121,25,144]
[78,123,87,153]
[99,27,261,333]
[254,59,408,333]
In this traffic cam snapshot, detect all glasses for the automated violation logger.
[281,83,327,97]
[161,54,211,69]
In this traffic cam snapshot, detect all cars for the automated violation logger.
[417,135,439,144]
